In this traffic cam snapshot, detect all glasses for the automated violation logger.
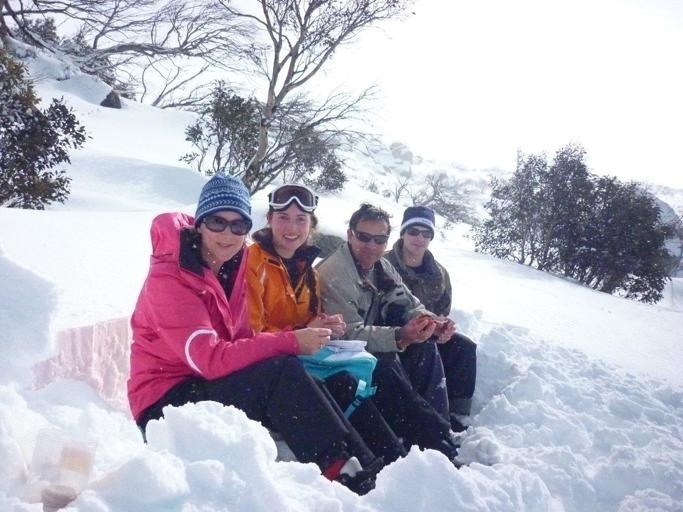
[267,185,320,212]
[405,226,433,239]
[200,215,251,235]
[350,228,389,244]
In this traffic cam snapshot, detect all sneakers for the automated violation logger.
[339,456,387,496]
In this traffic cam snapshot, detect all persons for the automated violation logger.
[125,174,385,495]
[385,203,477,433]
[247,183,405,464]
[315,201,464,468]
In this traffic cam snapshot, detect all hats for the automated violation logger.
[400,205,434,241]
[192,172,252,226]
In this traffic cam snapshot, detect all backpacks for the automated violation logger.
[295,338,377,389]
